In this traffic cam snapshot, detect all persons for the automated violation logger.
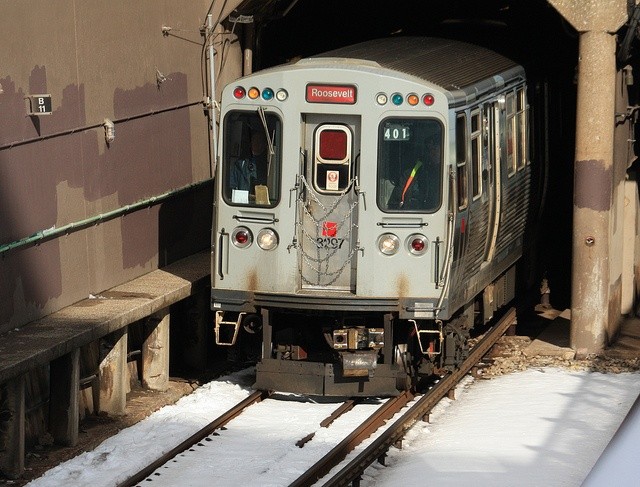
[230,132,268,195]
[387,133,442,210]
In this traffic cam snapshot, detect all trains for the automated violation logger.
[201,18,573,399]
